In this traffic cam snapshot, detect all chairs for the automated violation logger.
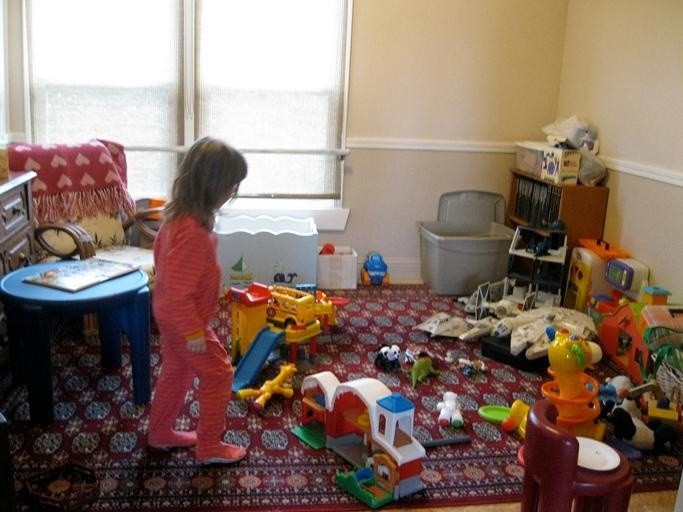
[8,139,168,348]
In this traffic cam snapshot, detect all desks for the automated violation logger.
[1,259,152,426]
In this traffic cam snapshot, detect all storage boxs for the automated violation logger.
[317,246,360,291]
[417,189,516,298]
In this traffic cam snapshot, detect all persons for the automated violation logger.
[147,137,247,465]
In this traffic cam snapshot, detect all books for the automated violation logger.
[514,178,561,227]
[22,258,141,292]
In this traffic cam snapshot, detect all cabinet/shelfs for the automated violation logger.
[0,167,40,401]
[505,167,609,295]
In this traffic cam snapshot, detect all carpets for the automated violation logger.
[1,285,683,512]
